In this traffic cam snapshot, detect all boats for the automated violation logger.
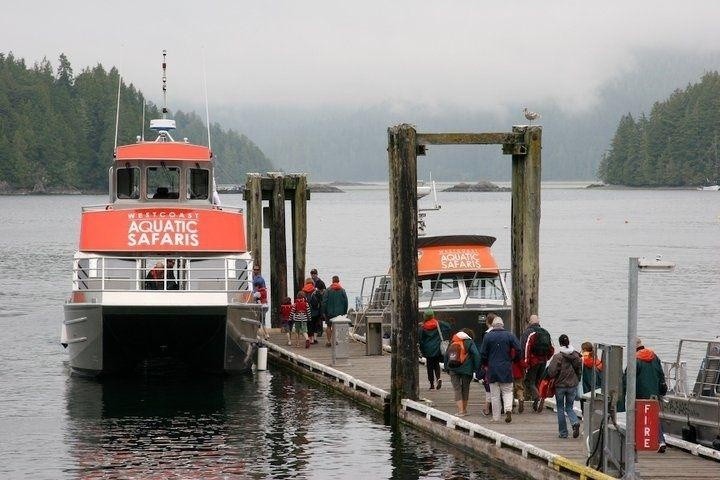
[638,257,677,272]
[579,330,720,454]
[697,184,720,191]
[345,172,513,366]
[63,43,268,381]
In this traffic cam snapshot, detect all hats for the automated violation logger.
[528,315,539,327]
[423,310,433,320]
[492,317,503,326]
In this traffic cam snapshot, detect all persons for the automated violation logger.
[417,310,451,390]
[546,334,581,439]
[277,269,348,349]
[142,259,179,289]
[444,327,482,413]
[253,282,270,340]
[474,313,554,424]
[622,337,667,452]
[251,264,266,293]
[578,342,603,436]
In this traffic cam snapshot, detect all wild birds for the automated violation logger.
[522,106,541,126]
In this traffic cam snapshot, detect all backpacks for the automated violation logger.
[448,335,466,367]
[532,328,551,356]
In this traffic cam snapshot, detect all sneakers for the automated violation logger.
[572,423,580,438]
[657,444,666,452]
[436,379,442,390]
[483,398,544,422]
[288,339,332,349]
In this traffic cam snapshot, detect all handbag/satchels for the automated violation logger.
[539,378,555,398]
[659,373,667,395]
[439,340,449,354]
[574,381,584,400]
